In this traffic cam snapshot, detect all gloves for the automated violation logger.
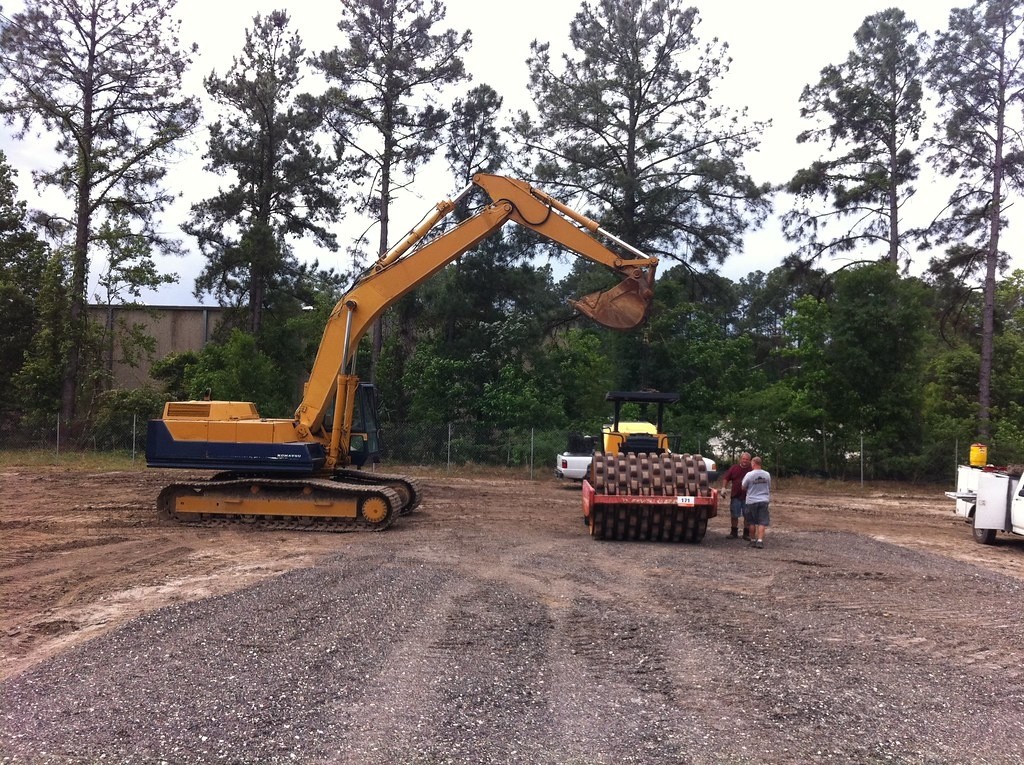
[720,487,728,499]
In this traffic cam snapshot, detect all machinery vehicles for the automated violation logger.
[583,391,719,543]
[145,173,660,531]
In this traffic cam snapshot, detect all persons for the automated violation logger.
[741,457,772,549]
[721,452,753,541]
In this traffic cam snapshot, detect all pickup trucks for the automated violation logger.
[554,448,719,483]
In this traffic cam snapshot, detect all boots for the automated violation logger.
[742,528,751,541]
[726,527,738,539]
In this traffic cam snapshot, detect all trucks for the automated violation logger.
[945,465,1024,544]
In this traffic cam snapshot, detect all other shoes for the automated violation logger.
[749,541,756,547]
[756,542,764,548]
